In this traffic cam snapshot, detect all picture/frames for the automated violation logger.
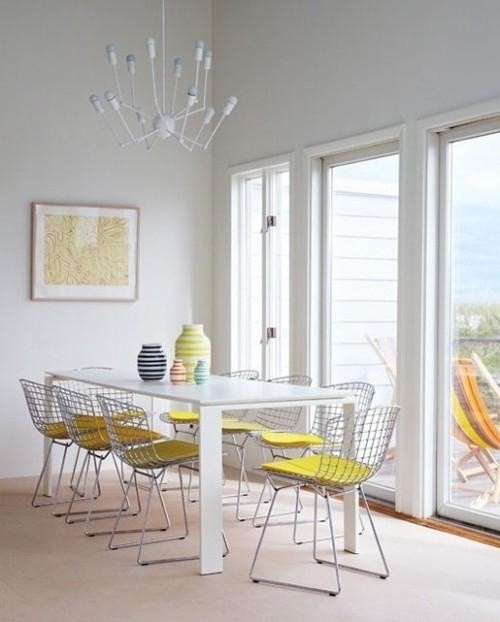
[29,200,144,305]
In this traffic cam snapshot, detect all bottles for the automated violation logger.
[137,342,167,382]
[175,323,212,384]
[194,358,211,386]
[169,359,187,386]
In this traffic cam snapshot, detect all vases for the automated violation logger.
[137,324,216,389]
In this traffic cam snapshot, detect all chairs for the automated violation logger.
[18,366,402,596]
[449,353,500,507]
[364,332,400,463]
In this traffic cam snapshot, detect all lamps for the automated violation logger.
[87,0,239,161]
